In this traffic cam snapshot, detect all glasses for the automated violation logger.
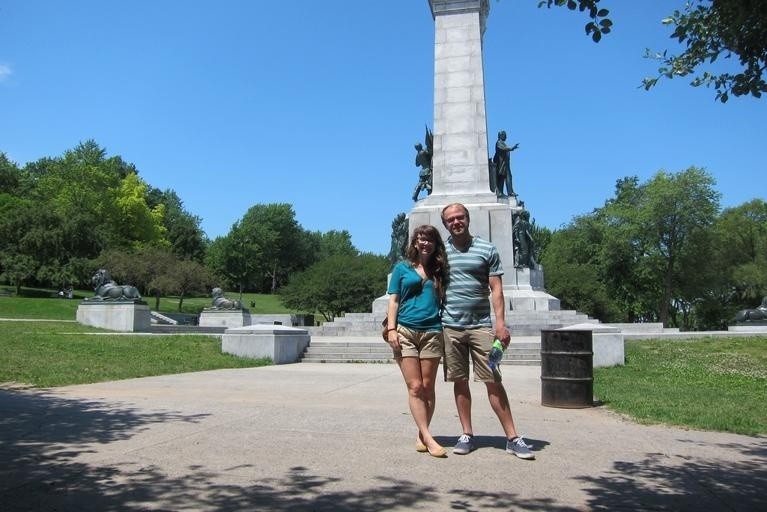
[416,237,435,245]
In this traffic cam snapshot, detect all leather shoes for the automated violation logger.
[415,444,427,452]
[428,446,447,457]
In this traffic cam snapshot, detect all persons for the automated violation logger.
[385,225,447,458]
[492,129,520,197]
[435,203,536,460]
[388,210,408,267]
[513,201,538,269]
[411,141,432,201]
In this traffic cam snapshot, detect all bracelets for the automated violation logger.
[386,328,395,332]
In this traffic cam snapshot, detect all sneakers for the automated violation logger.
[506,437,535,460]
[453,435,476,455]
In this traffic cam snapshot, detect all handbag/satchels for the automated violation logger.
[382,316,388,342]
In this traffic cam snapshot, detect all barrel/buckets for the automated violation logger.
[539,328,594,407]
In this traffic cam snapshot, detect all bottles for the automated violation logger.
[488,331,509,369]
